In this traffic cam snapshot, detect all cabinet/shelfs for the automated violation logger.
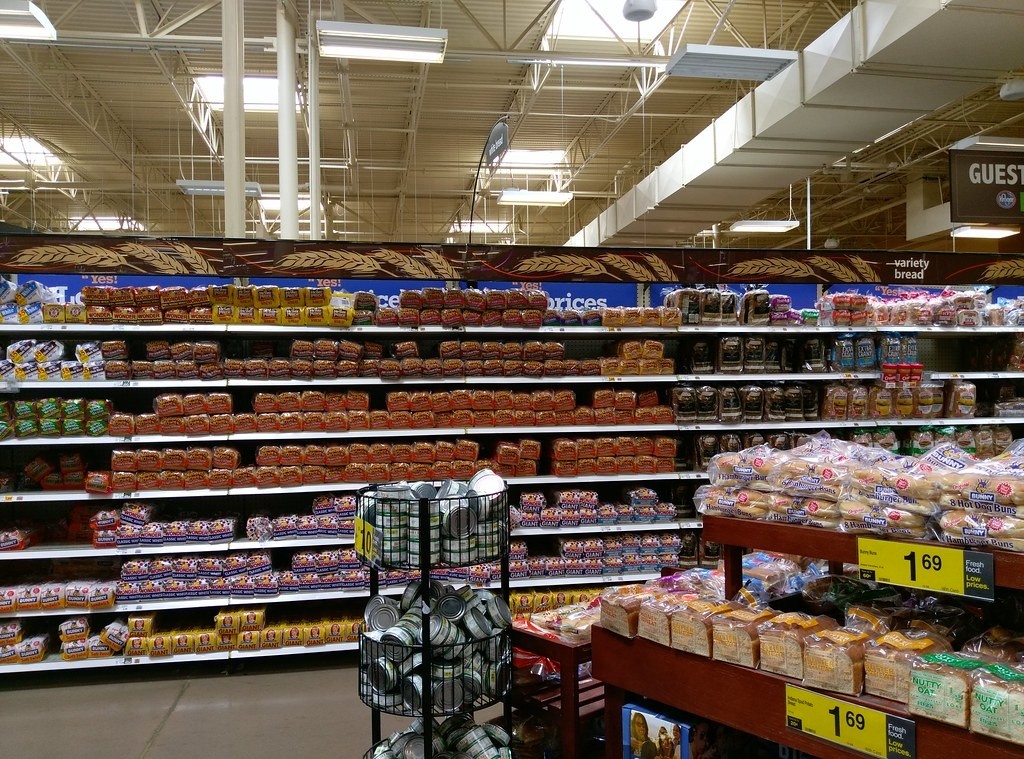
[590,514,1024,759]
[511,626,604,759]
[0,326,1024,673]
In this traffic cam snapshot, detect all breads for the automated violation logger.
[560,617,601,646]
[701,441,1024,550]
[601,550,1024,747]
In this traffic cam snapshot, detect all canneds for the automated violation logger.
[361,580,512,708]
[357,470,507,566]
[373,715,513,759]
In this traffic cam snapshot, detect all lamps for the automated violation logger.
[316,0,449,64]
[0,0,57,40]
[730,184,800,233]
[665,1,799,81]
[177,108,263,197]
[497,174,573,206]
[949,226,1020,239]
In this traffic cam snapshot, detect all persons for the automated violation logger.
[630,712,680,759]
